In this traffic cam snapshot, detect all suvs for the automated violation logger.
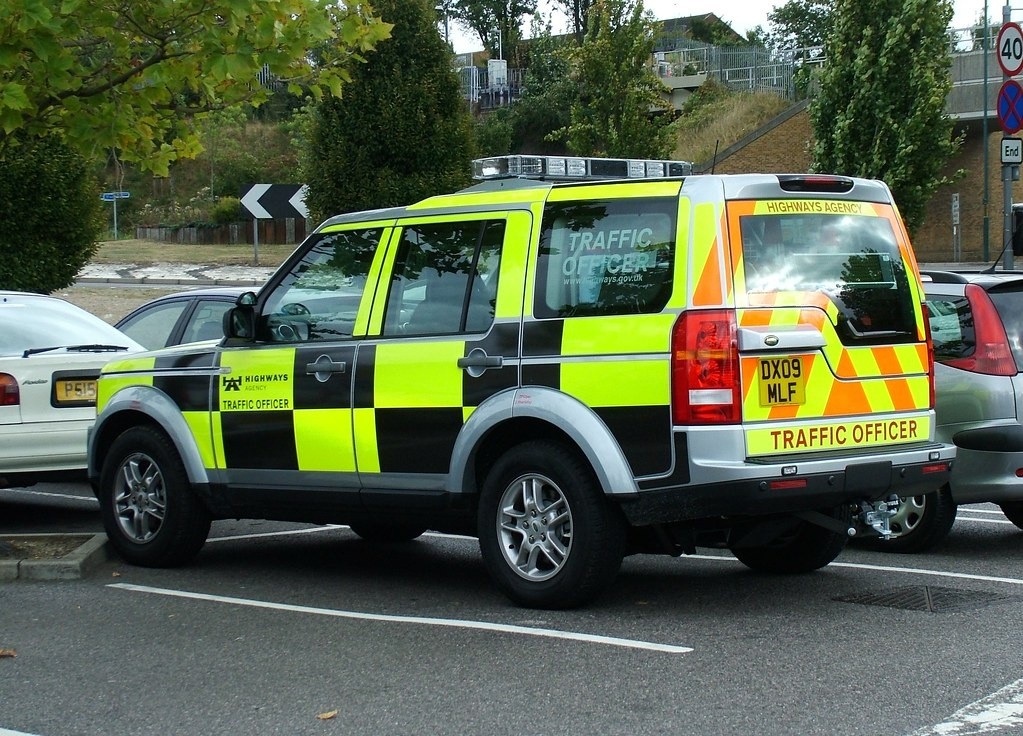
[751,269,1023,554]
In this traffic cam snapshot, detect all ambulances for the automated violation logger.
[85,154,961,615]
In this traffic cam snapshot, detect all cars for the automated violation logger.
[113,287,416,356]
[0,291,152,492]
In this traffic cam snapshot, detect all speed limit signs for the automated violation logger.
[997,21,1023,77]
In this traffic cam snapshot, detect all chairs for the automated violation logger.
[408,246,494,334]
[196,319,224,341]
